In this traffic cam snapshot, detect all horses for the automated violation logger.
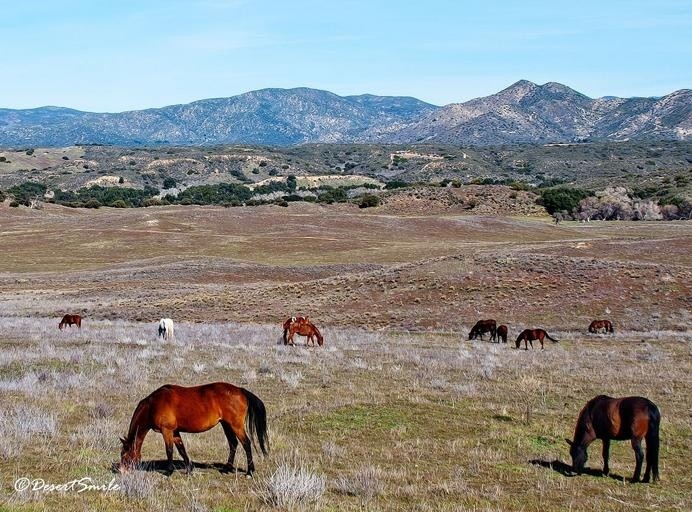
[588,319,614,334]
[118,380,272,479]
[157,317,175,341]
[58,313,82,331]
[564,393,662,485]
[281,315,326,347]
[466,318,510,344]
[515,328,561,350]
